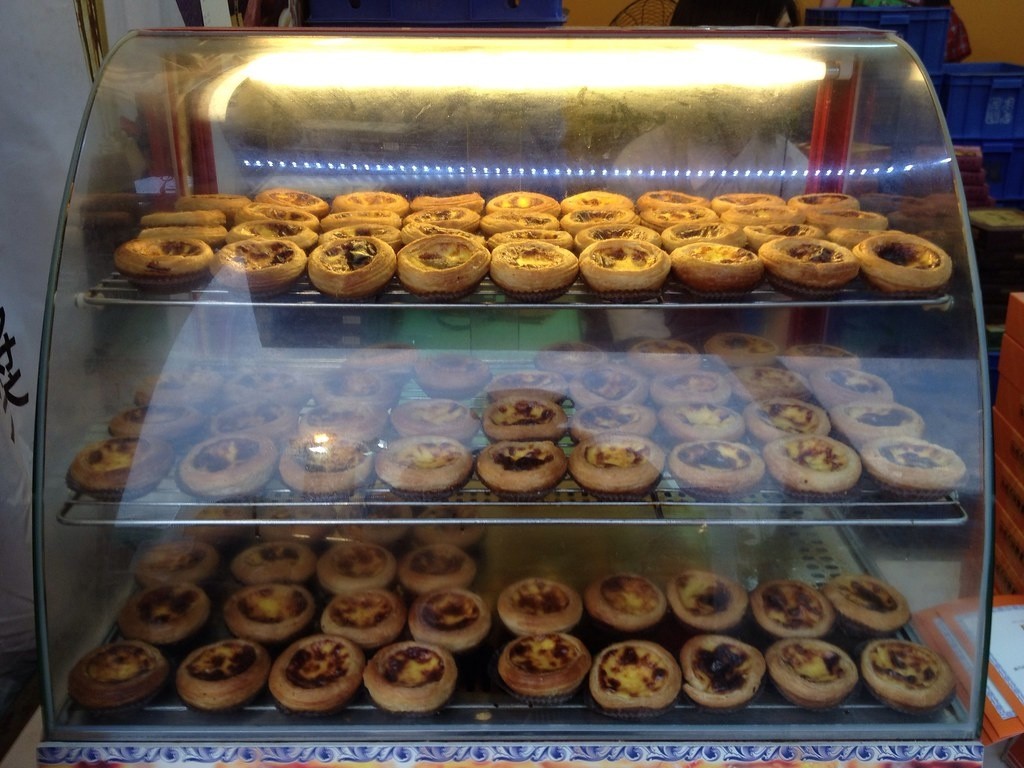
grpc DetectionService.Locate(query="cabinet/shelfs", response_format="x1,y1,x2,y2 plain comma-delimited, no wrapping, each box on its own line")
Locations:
35,27,994,739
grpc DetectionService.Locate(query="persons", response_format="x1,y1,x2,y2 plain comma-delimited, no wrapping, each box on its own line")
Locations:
600,1,809,351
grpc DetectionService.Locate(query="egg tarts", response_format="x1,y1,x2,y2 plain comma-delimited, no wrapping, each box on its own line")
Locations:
70,334,965,496
115,188,952,291
69,503,958,717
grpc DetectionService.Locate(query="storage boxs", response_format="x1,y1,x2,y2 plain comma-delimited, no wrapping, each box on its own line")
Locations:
958,292,1024,603
800,5,1024,203
302,0,568,29
